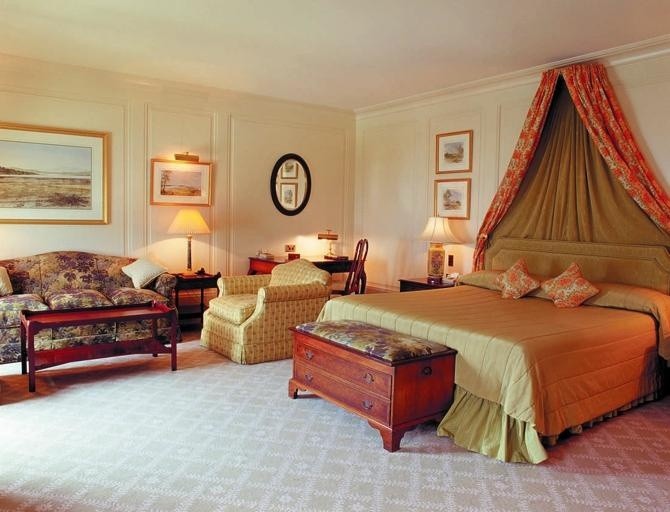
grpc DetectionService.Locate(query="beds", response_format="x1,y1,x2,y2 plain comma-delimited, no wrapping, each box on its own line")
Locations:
317,239,670,466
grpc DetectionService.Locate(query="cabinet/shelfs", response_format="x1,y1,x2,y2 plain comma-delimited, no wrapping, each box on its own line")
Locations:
288,320,458,453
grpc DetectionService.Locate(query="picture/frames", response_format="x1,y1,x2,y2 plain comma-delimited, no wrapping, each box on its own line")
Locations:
435,130,473,174
434,178,471,220
0,123,112,225
281,163,298,179
150,158,214,208
280,183,298,207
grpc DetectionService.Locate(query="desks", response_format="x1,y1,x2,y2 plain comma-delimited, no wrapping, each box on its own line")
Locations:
247,255,367,294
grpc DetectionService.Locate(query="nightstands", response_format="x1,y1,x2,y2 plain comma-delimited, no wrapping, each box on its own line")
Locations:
397,276,454,292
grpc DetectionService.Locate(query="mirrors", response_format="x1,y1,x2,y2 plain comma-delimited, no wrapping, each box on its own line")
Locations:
270,153,311,216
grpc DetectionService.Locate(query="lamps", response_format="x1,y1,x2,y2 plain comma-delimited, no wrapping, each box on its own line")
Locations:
415,217,464,284
317,229,338,259
166,209,212,271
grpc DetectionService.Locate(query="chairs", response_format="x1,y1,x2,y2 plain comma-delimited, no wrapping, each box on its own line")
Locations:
329,238,369,300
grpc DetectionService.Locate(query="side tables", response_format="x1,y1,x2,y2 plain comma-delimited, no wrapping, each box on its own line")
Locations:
170,267,221,330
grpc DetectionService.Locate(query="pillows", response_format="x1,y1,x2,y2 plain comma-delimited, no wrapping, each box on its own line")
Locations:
0,293,50,329
121,254,168,289
489,256,540,299
540,262,600,308
45,289,114,311
106,287,170,306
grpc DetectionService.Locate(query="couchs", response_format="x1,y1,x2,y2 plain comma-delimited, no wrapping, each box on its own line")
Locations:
200,259,333,365
0,251,182,365
